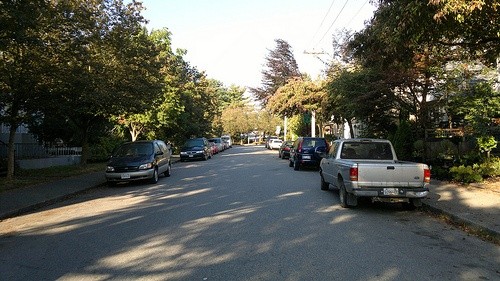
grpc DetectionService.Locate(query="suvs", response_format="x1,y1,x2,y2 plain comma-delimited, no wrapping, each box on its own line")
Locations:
179,137,212,162
289,137,331,170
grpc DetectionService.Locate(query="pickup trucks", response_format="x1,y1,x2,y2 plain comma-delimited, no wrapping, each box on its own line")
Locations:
319,138,431,208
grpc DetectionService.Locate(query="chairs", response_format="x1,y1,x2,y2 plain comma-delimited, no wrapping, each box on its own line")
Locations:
343,148,357,158
368,148,381,159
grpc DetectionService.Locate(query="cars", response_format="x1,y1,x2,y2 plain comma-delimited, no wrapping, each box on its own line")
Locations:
208,138,228,156
279,141,296,159
265,136,283,150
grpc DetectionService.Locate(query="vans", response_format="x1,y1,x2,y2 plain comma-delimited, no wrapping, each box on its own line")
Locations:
103,139,172,187
221,135,233,147
